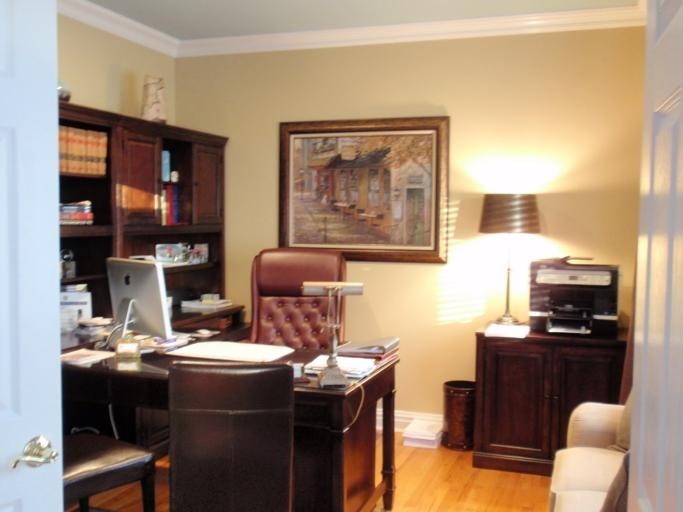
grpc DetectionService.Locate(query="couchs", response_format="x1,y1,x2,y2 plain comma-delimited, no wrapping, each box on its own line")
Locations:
546,387,628,512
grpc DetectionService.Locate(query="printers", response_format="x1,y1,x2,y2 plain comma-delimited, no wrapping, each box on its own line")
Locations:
529,261,619,334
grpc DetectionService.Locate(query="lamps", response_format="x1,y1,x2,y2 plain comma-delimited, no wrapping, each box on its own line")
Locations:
479,194,542,325
298,279,364,392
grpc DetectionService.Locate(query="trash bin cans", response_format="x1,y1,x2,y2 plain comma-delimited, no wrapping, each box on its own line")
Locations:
442,381,476,449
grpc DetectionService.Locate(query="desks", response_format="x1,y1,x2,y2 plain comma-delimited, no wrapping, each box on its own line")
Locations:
62,325,402,511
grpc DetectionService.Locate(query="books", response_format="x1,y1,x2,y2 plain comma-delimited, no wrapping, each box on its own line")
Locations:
59,127,183,226
303,327,400,379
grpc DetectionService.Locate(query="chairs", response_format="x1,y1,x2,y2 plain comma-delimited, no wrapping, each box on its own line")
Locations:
166,358,293,511
62,432,157,510
247,248,344,345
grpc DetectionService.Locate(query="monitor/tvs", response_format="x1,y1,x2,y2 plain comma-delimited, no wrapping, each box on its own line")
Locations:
106,257,172,341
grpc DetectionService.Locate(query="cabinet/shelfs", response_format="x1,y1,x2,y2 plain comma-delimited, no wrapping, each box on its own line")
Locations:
59,100,247,341
472,332,626,478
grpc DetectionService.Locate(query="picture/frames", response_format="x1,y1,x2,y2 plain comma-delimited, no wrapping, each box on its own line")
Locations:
276,115,450,264
